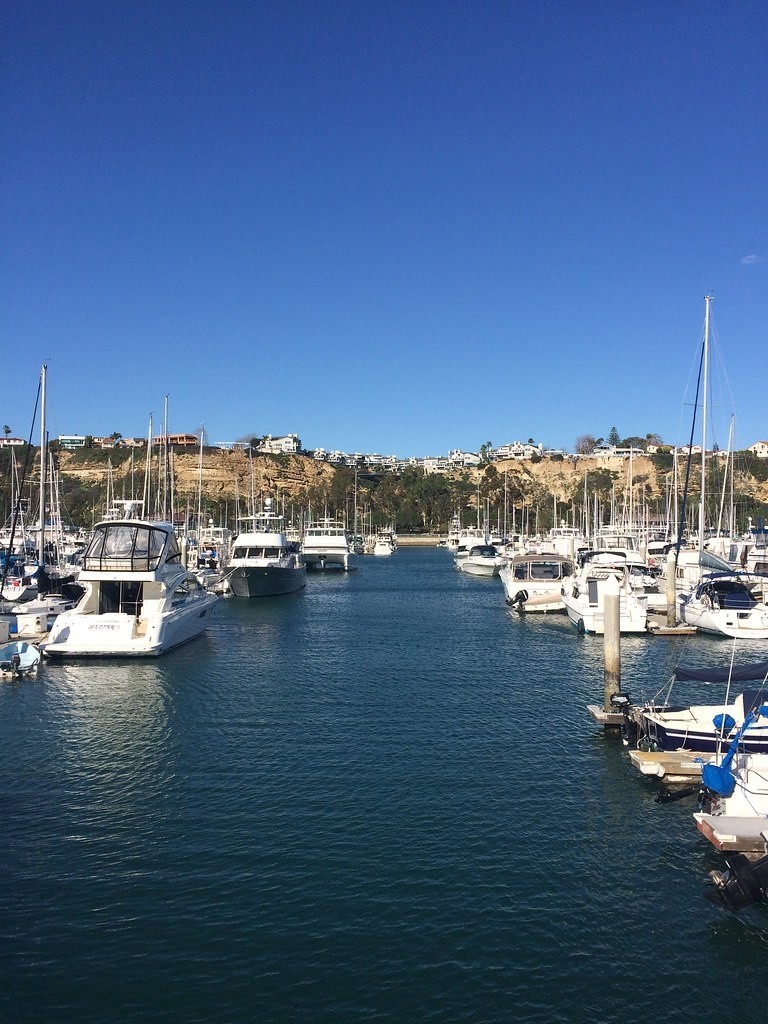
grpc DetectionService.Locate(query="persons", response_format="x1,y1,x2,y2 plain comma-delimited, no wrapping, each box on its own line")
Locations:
7,653,20,667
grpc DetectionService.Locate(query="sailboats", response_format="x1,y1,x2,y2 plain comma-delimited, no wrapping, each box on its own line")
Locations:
439,290,767,910
0,363,398,682
